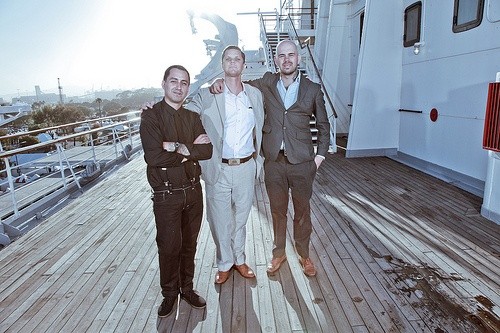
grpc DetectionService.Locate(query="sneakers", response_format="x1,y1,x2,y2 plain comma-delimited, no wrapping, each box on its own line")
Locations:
180,290,206,308
158,295,178,317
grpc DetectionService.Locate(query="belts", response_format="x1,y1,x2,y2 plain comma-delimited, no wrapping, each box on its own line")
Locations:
280,150,284,154
222,154,253,165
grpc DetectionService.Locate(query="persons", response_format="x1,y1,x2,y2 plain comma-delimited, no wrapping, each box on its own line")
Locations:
210,40,330,278
139,66,212,316
141,46,266,283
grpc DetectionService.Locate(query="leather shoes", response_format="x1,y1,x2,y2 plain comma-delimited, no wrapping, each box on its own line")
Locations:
215,266,232,283
266,254,287,273
232,264,255,278
299,256,316,276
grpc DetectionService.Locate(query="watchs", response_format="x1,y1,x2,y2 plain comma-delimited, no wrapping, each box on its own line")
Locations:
174,141,180,154
